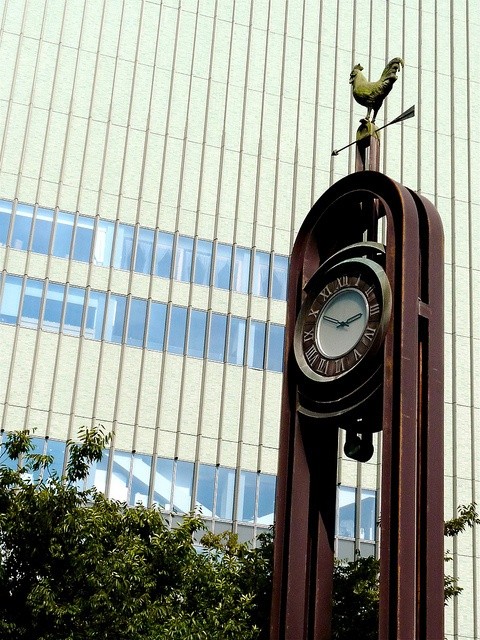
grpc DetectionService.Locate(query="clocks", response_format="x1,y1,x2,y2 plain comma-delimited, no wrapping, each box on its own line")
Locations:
293,256,393,384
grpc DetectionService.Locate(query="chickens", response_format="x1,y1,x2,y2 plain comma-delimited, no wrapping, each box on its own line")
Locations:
348,57,405,125
